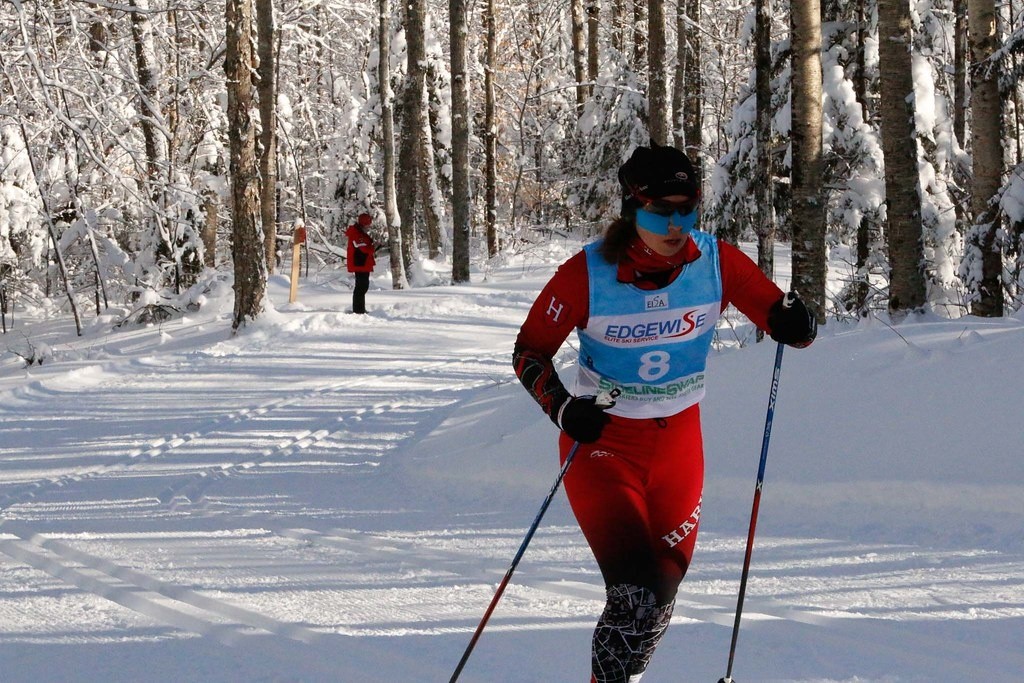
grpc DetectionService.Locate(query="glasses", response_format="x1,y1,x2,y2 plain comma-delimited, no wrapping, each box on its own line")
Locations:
638,198,699,217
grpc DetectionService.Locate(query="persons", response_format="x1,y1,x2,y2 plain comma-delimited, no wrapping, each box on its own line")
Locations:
512,137,818,683
344,213,385,314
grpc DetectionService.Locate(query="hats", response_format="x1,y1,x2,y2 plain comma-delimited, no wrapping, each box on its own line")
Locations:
618,138,697,200
358,213,372,227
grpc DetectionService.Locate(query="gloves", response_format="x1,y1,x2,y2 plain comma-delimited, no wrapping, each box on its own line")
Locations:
768,291,818,350
562,395,617,445
